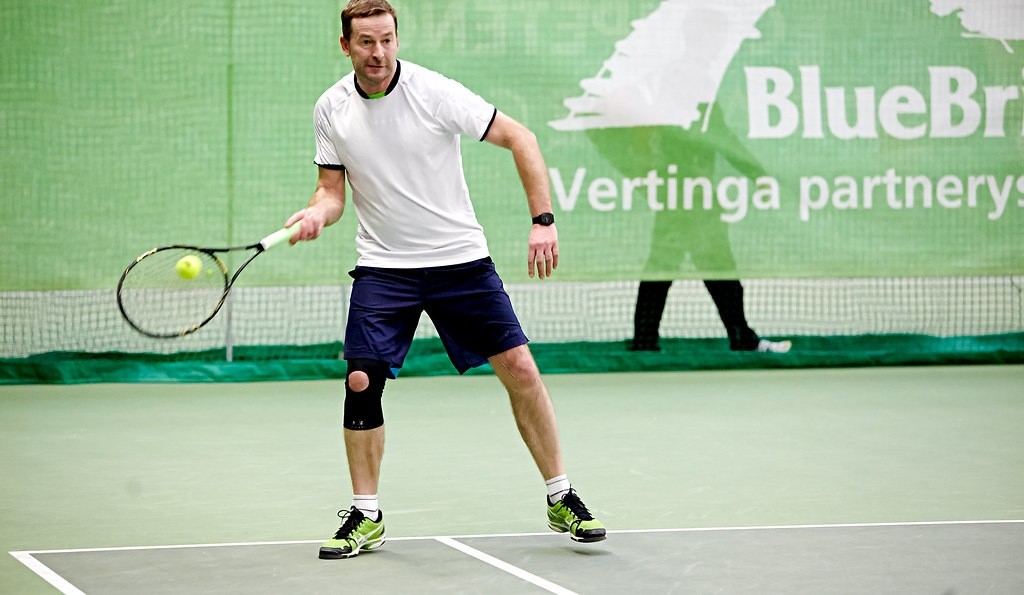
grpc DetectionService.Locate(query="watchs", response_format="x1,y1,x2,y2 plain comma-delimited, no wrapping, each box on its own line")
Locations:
532,211,555,226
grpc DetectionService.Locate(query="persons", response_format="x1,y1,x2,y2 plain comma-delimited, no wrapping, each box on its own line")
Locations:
626,102,793,352
279,1,607,560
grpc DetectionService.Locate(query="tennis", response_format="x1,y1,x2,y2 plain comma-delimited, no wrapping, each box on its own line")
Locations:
176,255,203,281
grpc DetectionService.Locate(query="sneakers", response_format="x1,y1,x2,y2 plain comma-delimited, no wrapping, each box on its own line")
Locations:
318,505,386,560
547,485,606,542
757,339,793,354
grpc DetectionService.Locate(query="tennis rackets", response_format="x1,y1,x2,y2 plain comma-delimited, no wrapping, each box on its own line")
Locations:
115,219,303,340
